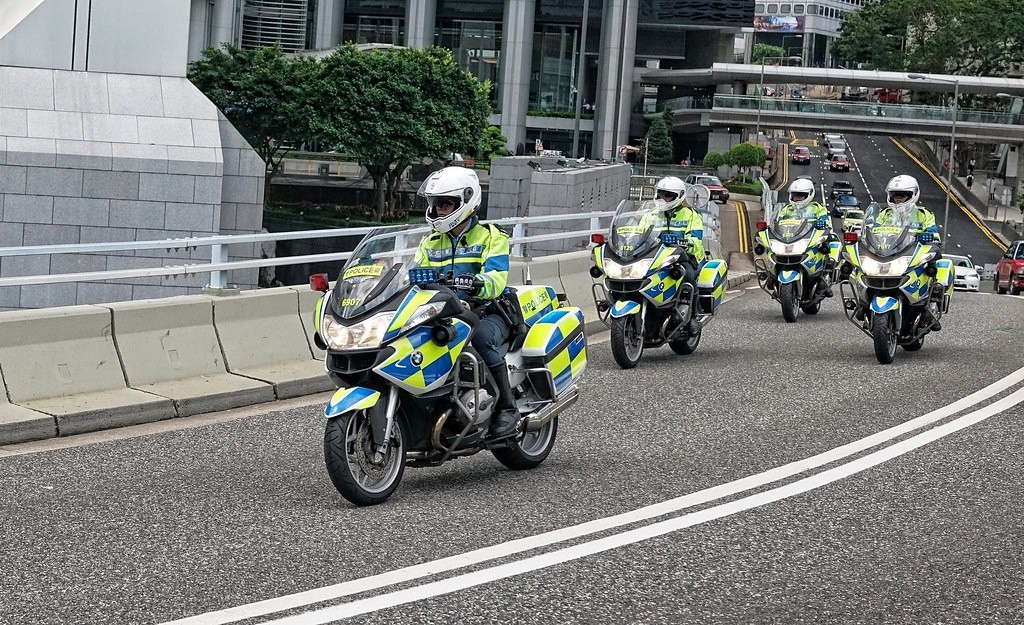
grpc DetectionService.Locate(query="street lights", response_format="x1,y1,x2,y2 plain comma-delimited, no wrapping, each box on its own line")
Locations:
784,45,809,99
776,34,802,98
908,73,961,253
753,55,802,181
885,33,905,106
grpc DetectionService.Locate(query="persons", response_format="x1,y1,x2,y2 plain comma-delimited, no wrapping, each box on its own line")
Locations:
873,174,941,332
771,178,832,298
637,176,704,333
764,87,767,95
990,177,995,200
945,160,950,169
779,89,783,96
966,173,973,189
970,157,975,171
681,157,697,166
413,165,521,437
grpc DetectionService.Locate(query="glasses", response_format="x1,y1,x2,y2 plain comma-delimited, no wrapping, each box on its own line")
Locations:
427,195,460,209
791,192,807,197
891,191,912,199
657,190,675,198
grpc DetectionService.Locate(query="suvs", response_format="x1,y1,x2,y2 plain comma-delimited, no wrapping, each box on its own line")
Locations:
993,239,1024,295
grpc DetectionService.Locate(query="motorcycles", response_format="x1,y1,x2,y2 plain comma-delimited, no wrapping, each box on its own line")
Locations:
588,198,728,369
839,201,955,364
752,201,844,323
307,223,589,508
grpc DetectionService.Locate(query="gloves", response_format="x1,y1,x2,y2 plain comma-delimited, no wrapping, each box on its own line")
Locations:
676,237,689,247
813,219,826,230
454,273,477,291
919,232,934,245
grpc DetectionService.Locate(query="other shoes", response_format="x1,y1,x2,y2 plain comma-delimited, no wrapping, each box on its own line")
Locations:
768,278,778,289
922,312,942,331
816,278,833,297
856,307,871,321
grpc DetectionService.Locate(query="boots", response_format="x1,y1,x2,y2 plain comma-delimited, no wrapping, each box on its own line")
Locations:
686,288,700,336
489,359,521,436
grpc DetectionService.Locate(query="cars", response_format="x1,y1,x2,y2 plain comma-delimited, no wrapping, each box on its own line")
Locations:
448,152,474,166
822,131,865,253
795,174,817,189
757,85,916,119
791,146,813,165
683,172,730,204
941,253,984,292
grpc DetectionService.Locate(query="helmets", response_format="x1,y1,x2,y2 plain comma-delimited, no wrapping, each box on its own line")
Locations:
417,166,481,234
654,177,686,212
884,175,921,213
787,179,815,209
